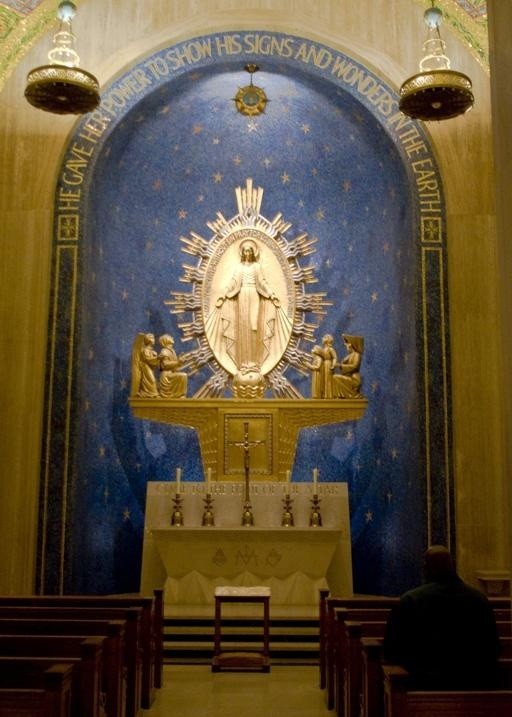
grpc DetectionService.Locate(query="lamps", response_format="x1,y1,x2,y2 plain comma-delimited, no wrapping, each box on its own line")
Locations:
23,0,101,118
396,0,476,122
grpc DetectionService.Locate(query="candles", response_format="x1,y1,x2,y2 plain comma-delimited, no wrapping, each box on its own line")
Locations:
285,467,291,495
314,465,317,494
176,466,180,494
207,466,212,494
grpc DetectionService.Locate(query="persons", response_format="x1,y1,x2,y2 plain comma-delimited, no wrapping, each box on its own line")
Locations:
323,334,337,398
205,245,294,370
384,546,504,691
137,333,165,399
234,441,261,470
332,333,364,398
158,334,188,398
304,345,324,399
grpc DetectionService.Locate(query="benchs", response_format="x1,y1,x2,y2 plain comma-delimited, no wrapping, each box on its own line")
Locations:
0,588,165,717
319,587,512,717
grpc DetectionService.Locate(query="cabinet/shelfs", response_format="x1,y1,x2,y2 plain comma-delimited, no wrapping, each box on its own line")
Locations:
211,586,271,673
